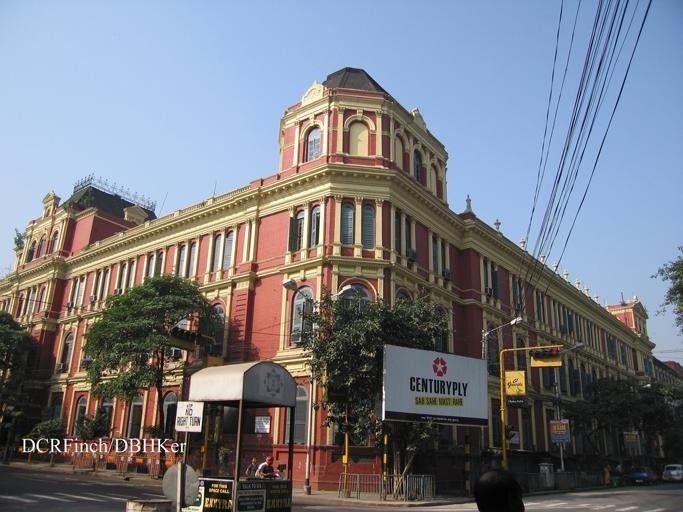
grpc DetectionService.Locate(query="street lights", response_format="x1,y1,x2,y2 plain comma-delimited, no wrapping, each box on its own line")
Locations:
480,316,524,448
282,278,356,486
554,342,586,472
630,383,651,394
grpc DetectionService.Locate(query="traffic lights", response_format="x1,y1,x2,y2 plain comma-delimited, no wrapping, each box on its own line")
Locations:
169,326,196,343
504,424,516,441
529,347,559,359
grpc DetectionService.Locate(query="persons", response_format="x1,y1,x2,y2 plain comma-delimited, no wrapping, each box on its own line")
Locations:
471,467,525,511
244,457,257,478
252,455,281,480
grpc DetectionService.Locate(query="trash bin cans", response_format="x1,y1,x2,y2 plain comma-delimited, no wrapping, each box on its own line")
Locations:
538,463,555,491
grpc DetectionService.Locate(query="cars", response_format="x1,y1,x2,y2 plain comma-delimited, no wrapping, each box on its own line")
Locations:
661,464,683,483
630,467,661,486
